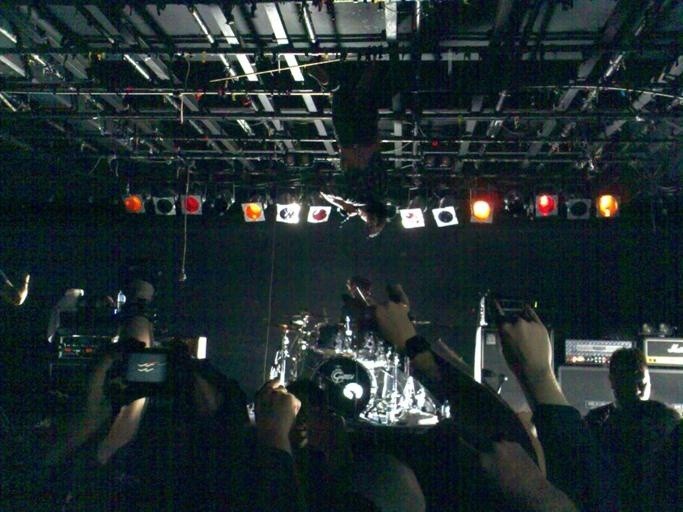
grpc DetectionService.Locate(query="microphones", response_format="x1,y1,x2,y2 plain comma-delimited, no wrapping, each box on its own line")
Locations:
497,375,509,395
334,373,354,381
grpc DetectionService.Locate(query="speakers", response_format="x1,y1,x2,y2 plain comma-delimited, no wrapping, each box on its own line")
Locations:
557,364,614,418
649,369,683,408
473,326,555,416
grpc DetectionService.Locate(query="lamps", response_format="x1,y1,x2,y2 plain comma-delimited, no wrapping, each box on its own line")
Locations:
120,182,336,228
394,186,626,232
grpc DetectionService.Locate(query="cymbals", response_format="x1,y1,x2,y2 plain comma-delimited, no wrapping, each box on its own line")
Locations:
264,319,302,331
409,312,433,327
281,312,330,318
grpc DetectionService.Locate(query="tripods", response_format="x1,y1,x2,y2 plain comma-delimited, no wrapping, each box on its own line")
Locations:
361,347,437,421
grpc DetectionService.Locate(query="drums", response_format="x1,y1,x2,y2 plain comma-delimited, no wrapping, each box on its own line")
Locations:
313,322,347,357
309,353,379,418
302,349,328,381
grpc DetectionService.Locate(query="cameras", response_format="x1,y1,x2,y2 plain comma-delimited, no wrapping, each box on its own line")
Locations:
121,348,174,386
